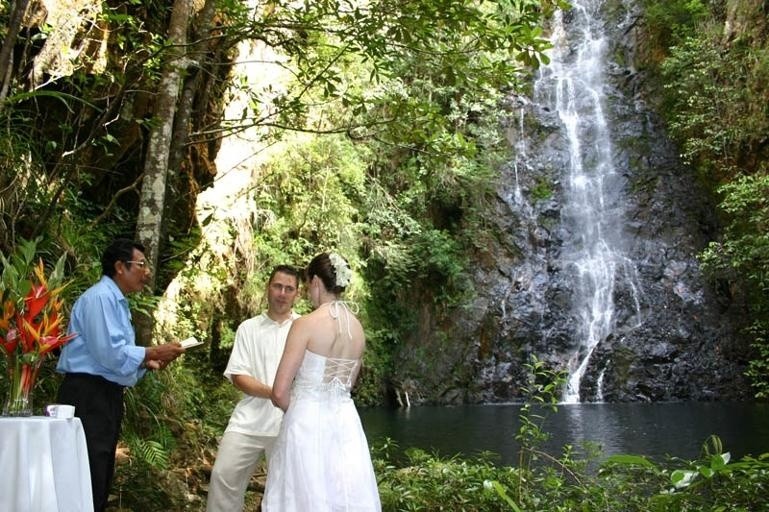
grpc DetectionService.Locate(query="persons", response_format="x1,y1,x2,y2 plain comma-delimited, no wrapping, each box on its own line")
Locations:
261,252,383,511
53,242,185,512
206,267,301,512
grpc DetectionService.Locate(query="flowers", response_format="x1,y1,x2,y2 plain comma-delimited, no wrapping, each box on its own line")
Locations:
0,232,81,409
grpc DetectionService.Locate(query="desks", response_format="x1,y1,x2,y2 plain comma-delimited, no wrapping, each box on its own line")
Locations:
0,415,95,512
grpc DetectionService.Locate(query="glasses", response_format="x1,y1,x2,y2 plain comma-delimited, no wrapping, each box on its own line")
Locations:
126,260,151,269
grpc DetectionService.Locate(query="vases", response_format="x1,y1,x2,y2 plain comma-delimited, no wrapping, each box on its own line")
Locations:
4,350,43,417
